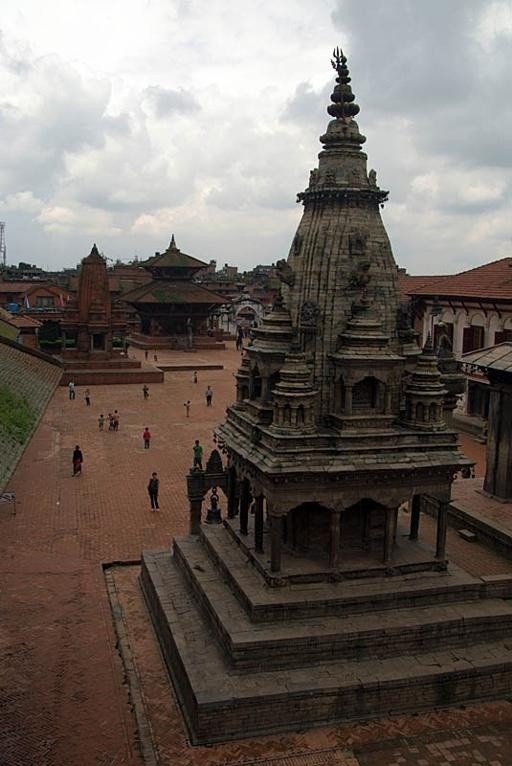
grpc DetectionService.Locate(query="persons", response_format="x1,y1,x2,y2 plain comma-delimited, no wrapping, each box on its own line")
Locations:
84,388,91,408
194,371,198,383
68,379,76,400
147,472,160,512
143,385,149,400
145,349,149,361
192,440,204,470
98,410,120,431
143,427,151,448
154,349,158,361
205,385,213,406
72,445,83,477
